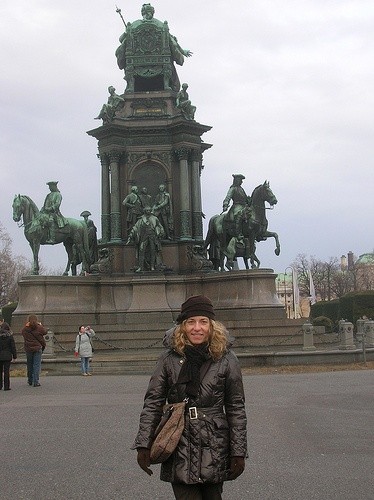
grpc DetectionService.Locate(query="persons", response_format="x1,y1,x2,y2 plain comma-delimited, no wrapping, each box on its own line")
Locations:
93,86,124,120
151,184,174,241
223,174,249,239
121,185,143,234
22,314,47,387
74,325,98,376
139,188,153,207
114,3,193,92
179,83,196,118
130,295,248,500
126,206,165,272
80,211,97,263
0,322,18,390
40,181,62,246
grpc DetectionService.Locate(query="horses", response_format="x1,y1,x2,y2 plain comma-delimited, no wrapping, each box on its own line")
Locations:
12,194,92,277
201,180,282,273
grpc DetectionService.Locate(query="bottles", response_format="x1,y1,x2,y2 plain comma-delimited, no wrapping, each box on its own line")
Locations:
84,327,89,330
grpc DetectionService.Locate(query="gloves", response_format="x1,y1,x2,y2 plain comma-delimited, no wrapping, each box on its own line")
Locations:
74,352,78,358
13,354,17,359
137,448,153,476
86,326,90,330
229,456,245,480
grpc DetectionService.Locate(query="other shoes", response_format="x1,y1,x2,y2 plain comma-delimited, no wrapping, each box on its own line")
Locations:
87,372,92,376
34,382,40,386
4,388,11,390
29,382,32,385
82,372,87,376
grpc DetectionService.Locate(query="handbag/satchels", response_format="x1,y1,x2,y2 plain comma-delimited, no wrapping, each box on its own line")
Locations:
42,346,45,353
92,348,94,352
149,403,187,464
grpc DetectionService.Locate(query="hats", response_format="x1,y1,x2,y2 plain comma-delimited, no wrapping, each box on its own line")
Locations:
181,295,215,322
